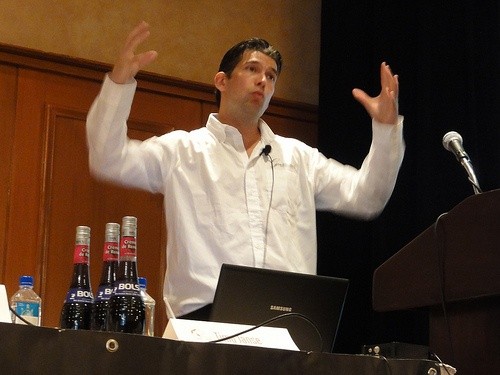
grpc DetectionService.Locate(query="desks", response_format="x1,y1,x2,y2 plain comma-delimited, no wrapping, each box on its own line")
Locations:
0,322,441,375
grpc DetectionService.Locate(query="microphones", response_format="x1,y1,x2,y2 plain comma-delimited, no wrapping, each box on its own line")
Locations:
261,145,272,155
443,131,471,164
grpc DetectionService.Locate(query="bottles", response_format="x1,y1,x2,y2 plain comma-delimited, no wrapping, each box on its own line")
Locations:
103,216,147,335
138,277,156,337
60,225,94,330
90,223,120,330
9,275,43,327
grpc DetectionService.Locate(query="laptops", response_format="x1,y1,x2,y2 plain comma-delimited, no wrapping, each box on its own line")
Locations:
207,263,351,354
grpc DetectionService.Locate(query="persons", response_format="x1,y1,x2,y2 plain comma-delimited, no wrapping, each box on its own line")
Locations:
86,19,404,327
